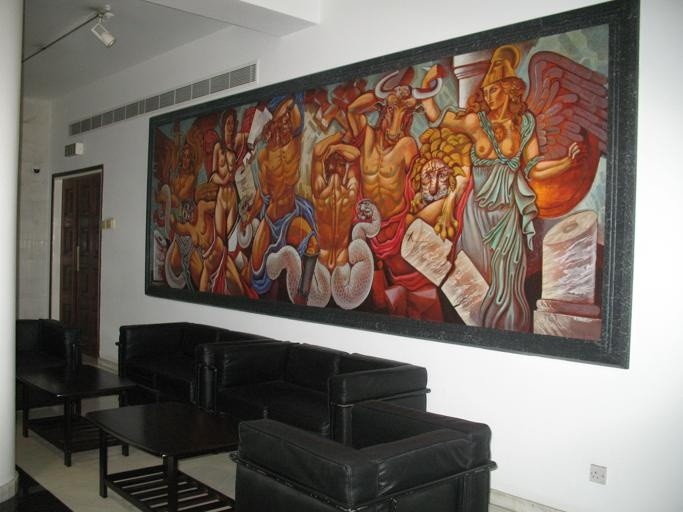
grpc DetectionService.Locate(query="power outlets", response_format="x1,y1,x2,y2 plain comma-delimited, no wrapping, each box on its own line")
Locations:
590,464,607,485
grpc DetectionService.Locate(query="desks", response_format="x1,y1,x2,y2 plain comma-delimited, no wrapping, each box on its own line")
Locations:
87,402,240,512
16,364,139,468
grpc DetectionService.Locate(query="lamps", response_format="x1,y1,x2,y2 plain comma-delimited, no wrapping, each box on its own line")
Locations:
92,6,116,48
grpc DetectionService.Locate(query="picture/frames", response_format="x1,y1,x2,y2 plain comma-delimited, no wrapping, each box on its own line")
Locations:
144,0,640,369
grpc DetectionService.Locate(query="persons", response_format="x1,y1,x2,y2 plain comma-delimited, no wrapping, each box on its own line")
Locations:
151,41,583,339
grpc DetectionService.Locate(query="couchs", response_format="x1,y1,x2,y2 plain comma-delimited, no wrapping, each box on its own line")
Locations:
115,322,434,452
15,319,84,409
228,397,499,512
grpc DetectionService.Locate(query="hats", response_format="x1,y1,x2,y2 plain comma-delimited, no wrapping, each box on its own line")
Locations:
480,56,517,90
